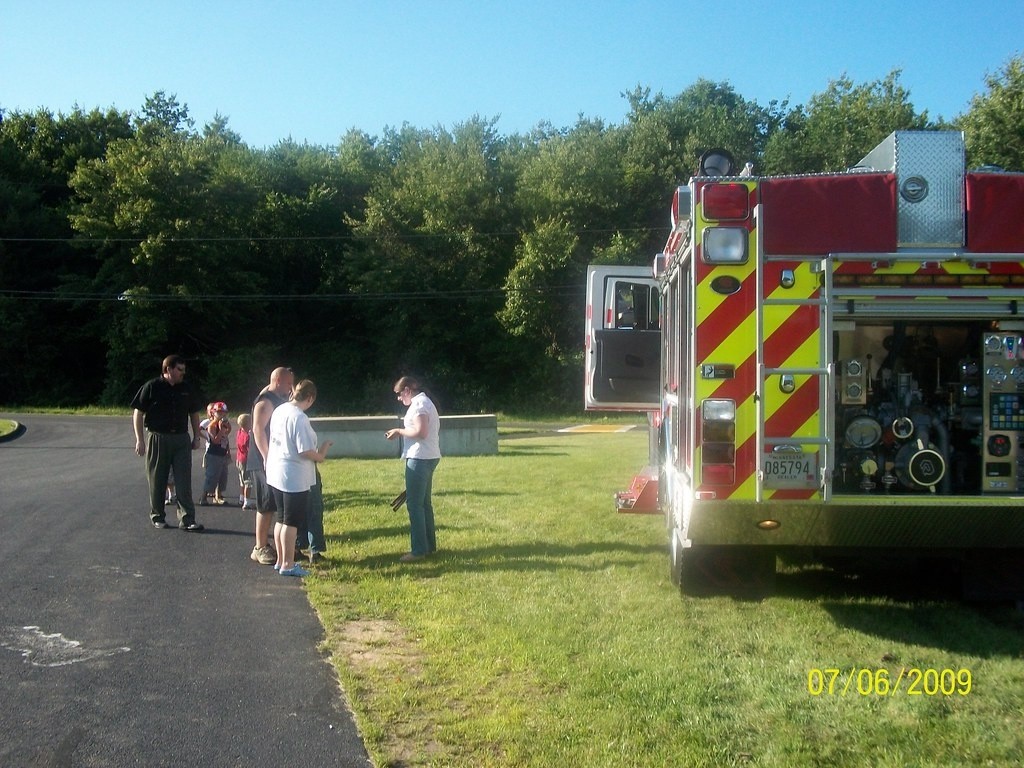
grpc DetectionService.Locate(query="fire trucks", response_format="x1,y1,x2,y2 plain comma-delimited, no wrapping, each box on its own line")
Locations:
583,133,1024,610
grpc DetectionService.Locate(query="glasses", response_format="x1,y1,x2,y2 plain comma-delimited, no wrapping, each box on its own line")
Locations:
394,389,405,397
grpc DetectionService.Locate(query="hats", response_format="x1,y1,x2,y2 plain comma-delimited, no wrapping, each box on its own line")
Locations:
213,401,228,412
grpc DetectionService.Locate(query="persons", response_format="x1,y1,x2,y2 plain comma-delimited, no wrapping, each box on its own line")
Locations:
199,402,232,505
165,462,176,505
235,414,257,509
245,367,294,564
199,403,215,497
384,376,442,561
130,356,205,532
264,378,324,576
294,440,335,563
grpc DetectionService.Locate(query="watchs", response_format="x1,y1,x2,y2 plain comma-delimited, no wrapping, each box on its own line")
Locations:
194,438,200,440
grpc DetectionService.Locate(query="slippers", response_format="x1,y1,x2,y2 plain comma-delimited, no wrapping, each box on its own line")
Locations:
274,562,302,570
279,566,310,576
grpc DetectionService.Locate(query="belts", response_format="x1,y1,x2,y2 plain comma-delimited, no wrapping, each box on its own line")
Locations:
148,429,187,434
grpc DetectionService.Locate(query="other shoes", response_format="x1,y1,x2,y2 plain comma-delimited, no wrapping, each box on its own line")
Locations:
239,500,244,505
294,551,309,562
310,553,325,564
426,552,431,555
212,497,226,505
242,503,257,510
168,497,175,504
400,553,425,561
199,498,208,505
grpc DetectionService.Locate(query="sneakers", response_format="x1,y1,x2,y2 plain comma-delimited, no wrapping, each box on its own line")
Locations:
250,544,276,564
178,520,204,532
149,509,169,529
